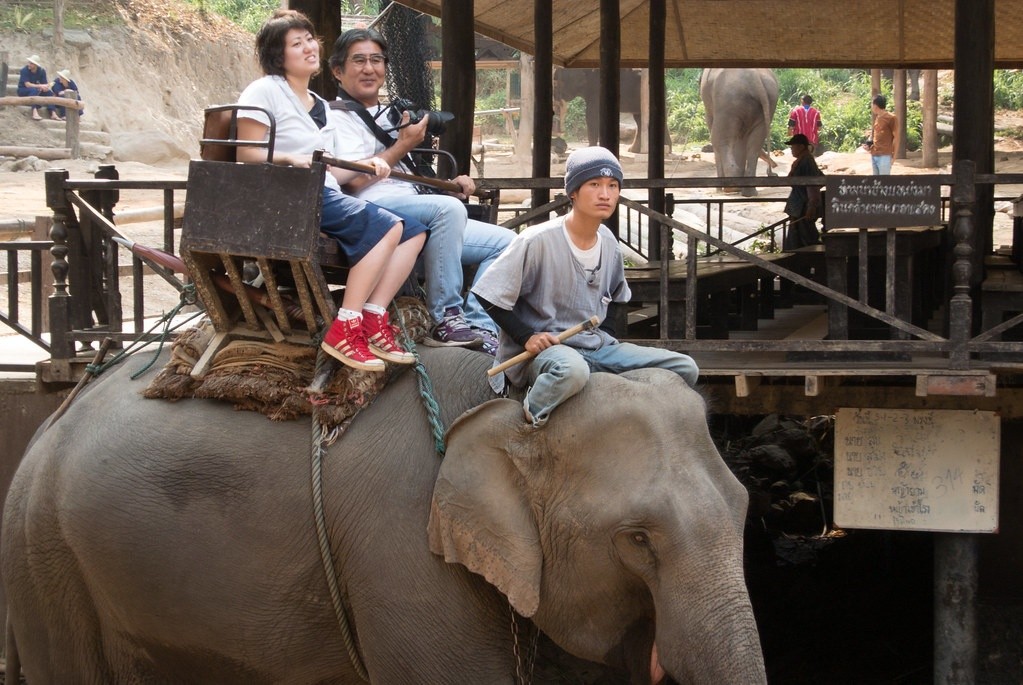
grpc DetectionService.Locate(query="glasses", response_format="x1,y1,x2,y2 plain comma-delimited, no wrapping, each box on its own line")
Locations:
347,54,386,66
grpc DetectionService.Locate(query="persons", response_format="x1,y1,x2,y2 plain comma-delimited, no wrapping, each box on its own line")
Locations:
17,55,62,121
235,10,431,371
52,69,84,123
322,27,519,356
783,133,826,252
788,95,823,157
470,146,699,428
863,94,900,176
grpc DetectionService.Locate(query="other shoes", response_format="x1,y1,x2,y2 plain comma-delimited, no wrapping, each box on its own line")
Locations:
32,115,42,120
51,116,62,121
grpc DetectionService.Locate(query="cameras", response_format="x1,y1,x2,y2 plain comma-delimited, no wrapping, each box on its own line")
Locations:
386,98,454,137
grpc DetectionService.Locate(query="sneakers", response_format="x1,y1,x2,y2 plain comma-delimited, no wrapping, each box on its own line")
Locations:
362,311,415,364
321,316,385,371
471,327,499,356
424,306,484,349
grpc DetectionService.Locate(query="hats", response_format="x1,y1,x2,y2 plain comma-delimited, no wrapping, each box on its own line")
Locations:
57,70,72,83
564,146,624,201
27,55,41,67
783,135,810,146
873,95,887,110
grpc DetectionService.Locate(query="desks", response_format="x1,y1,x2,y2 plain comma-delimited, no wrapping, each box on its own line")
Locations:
782,245,829,305
980,269,1023,342
678,252,797,320
984,254,1017,270
787,225,946,364
621,262,759,340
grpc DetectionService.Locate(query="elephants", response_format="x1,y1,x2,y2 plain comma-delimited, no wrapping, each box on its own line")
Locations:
554,66,674,155
699,67,779,199
1,328,771,685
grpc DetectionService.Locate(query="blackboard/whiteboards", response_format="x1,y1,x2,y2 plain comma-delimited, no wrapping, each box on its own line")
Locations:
833,407,1001,533
824,176,941,231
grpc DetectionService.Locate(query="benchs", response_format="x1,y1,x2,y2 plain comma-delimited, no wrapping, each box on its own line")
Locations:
180,104,501,269
782,312,828,340
627,305,659,332
